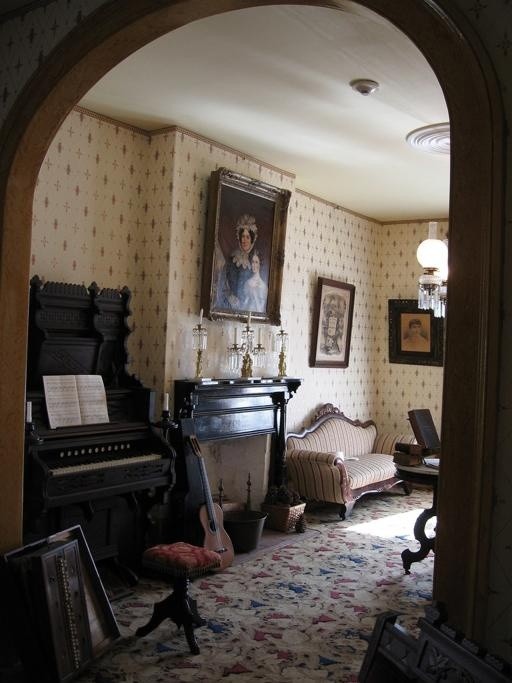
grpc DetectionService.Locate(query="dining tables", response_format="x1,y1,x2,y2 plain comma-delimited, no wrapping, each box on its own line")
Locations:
260,483,307,532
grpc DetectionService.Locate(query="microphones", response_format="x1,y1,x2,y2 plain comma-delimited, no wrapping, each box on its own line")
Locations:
134,541,222,656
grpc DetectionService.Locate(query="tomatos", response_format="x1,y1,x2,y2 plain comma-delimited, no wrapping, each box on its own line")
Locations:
23,274,176,588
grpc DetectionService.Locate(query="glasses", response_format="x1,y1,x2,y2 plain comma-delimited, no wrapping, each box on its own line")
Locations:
417,220,448,319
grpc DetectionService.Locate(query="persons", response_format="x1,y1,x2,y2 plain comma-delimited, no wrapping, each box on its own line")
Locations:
212,212,267,314
399,317,430,352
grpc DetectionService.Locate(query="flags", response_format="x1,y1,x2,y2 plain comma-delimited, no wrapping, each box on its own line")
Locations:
223,509,268,554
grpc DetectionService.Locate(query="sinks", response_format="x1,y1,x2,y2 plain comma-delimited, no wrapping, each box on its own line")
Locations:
285,403,419,520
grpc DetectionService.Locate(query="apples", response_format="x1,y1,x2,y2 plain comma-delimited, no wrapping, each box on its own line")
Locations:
163,392,169,411
26,401,32,422
199,308,204,327
280,320,284,329
247,310,252,330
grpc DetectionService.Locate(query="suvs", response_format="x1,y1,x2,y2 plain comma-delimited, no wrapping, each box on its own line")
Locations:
222,509,269,555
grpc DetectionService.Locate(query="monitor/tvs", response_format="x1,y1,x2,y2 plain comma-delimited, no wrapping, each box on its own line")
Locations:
42,374,110,430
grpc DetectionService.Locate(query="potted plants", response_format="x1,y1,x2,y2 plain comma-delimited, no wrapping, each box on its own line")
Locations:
388,298,444,367
200,166,292,327
309,276,356,368
